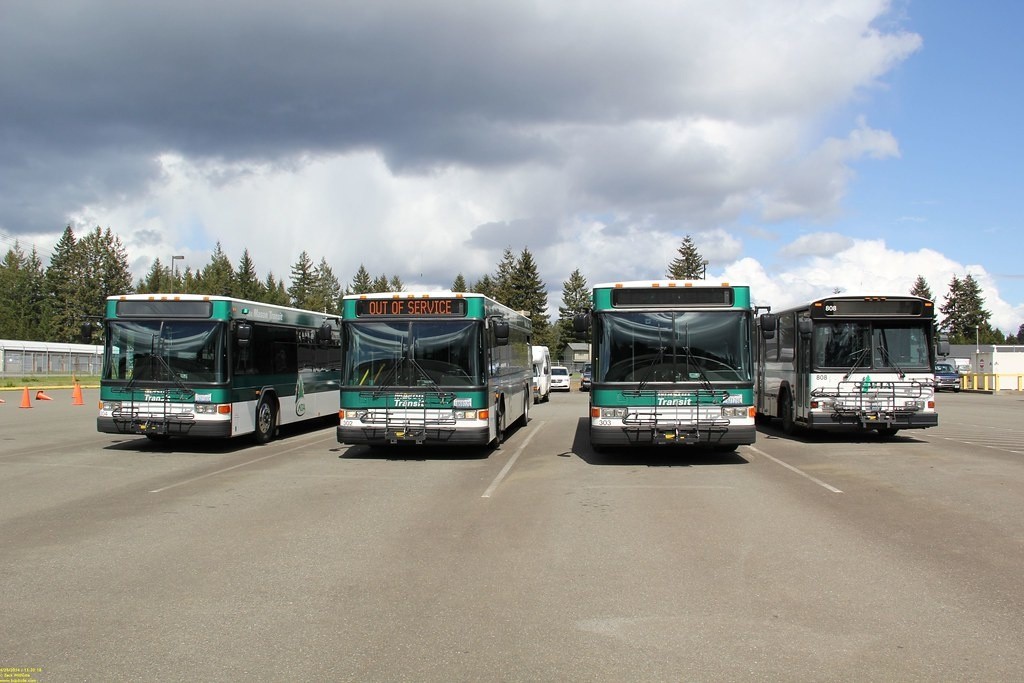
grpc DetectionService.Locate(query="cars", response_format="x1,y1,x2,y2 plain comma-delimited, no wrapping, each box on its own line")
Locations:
551,367,573,393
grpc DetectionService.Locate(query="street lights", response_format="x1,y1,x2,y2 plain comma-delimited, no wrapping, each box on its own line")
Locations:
170,255,184,294
701,259,709,280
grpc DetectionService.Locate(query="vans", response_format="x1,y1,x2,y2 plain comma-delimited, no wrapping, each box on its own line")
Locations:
530,346,553,403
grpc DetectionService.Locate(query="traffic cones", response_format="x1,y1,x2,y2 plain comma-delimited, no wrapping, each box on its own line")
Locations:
18,385,32,408
72,383,78,398
0,399,5,403
72,385,85,404
36,390,53,400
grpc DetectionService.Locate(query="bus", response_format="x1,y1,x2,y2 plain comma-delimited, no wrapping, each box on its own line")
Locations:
97,293,341,444
336,291,534,448
749,296,950,439
571,280,778,458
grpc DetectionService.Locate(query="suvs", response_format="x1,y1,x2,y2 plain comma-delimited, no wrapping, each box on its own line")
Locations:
934,364,960,393
580,361,592,391
